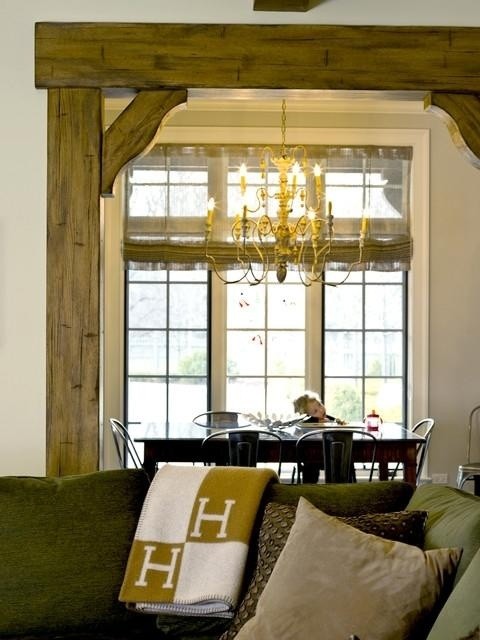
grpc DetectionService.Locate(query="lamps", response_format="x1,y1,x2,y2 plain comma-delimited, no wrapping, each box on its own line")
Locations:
202,98,368,286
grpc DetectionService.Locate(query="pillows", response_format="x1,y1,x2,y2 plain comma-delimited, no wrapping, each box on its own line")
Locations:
219,496,463,640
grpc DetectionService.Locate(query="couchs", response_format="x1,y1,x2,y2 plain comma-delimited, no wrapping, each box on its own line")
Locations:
1,470,479,640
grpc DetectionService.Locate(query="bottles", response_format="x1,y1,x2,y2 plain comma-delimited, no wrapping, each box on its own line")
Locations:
367,409,381,432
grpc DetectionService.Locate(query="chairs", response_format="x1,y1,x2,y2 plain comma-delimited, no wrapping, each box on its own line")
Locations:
458,405,480,491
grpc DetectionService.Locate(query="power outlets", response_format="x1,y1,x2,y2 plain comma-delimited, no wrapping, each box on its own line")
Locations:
433,474,448,484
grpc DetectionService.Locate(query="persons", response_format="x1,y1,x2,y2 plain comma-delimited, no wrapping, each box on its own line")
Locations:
295,391,358,485
